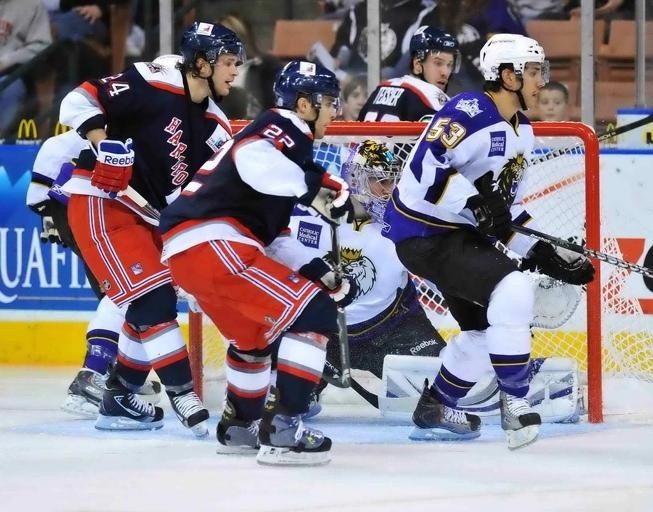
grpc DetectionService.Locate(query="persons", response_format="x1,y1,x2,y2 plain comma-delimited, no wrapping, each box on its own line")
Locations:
264,140,447,420
26,54,185,408
47,20,245,429
380,33,595,435
1,2,629,142
159,58,350,451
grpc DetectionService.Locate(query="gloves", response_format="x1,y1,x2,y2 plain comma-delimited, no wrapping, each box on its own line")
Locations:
527,240,595,284
301,257,358,306
301,166,354,225
89,138,135,198
474,171,512,243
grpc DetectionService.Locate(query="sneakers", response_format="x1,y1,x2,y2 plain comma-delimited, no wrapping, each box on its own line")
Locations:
260,408,332,451
413,378,481,433
216,417,259,450
66,368,164,422
167,389,209,427
498,390,540,431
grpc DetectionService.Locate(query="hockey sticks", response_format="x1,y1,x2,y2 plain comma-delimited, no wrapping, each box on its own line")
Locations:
322,190,353,387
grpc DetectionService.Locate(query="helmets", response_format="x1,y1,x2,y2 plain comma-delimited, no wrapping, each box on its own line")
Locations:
273,60,340,135
179,20,248,103
480,34,551,111
409,26,462,94
352,140,403,219
